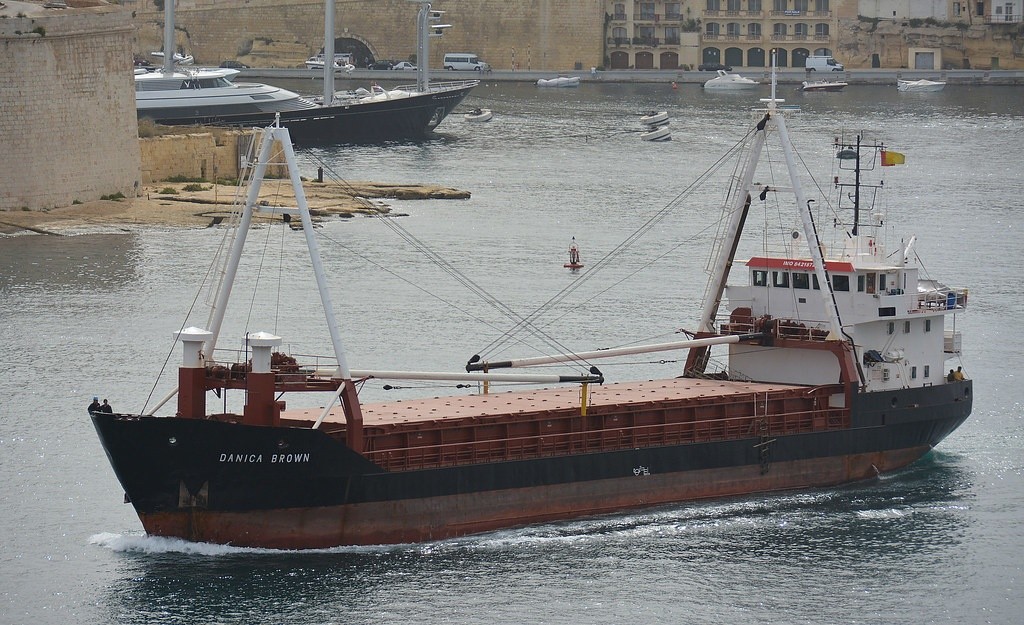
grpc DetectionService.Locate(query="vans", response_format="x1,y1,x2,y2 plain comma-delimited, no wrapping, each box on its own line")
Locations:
443,52,486,71
805,54,843,73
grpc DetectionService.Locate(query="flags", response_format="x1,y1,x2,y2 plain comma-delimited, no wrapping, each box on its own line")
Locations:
880,150,905,165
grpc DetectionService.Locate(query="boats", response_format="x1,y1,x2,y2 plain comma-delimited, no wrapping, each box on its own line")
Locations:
85,44,974,552
800,80,849,91
537,75,580,87
126,0,241,90
133,1,481,143
896,78,946,92
703,68,760,92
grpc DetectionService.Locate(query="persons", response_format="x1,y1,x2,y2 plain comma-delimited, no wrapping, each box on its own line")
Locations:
953,365,965,383
87,396,112,413
947,368,955,382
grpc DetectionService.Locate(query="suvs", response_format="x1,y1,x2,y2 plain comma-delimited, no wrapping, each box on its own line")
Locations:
305,60,355,77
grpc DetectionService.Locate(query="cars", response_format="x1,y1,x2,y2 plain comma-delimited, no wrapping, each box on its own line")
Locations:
697,61,733,72
393,62,421,72
133,58,149,66
368,59,396,71
218,60,250,69
